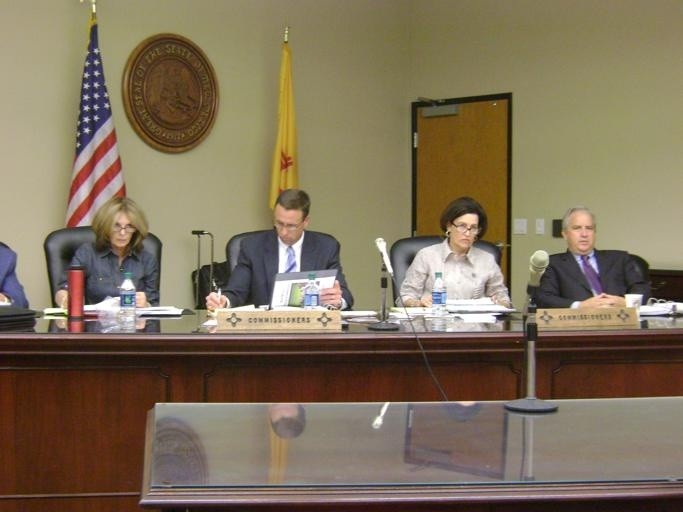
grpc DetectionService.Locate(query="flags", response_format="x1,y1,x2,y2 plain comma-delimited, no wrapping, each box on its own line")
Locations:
269,44,297,209
65,15,129,229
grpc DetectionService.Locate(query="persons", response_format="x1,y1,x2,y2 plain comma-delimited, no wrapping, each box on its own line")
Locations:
537,207,651,309
0,243,30,307
205,190,353,313
396,197,510,307
54,197,160,308
266,403,305,440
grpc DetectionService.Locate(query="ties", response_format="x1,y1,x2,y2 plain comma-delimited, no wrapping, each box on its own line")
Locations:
284,247,296,273
581,255,602,296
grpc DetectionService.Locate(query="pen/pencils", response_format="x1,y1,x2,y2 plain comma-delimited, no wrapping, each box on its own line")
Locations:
216,288,221,303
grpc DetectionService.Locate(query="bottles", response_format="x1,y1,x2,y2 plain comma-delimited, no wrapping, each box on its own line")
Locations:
119,273,137,330
431,272,447,326
67,266,87,319
303,273,321,312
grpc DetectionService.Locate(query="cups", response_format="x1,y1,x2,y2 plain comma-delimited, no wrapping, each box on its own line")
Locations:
624,293,644,319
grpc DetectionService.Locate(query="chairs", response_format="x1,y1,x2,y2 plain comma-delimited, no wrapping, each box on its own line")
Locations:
630,255,650,274
390,235,503,308
227,230,273,280
44,226,163,306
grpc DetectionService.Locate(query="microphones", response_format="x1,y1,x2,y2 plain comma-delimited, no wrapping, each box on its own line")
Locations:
374,237,395,276
522,251,550,302
371,402,390,430
191,230,214,238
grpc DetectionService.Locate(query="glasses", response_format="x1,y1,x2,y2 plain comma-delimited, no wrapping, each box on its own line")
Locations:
450,222,482,236
270,219,302,231
109,225,137,233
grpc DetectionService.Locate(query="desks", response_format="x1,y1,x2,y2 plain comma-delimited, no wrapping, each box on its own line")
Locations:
140,395,682,512
2,296,682,512
